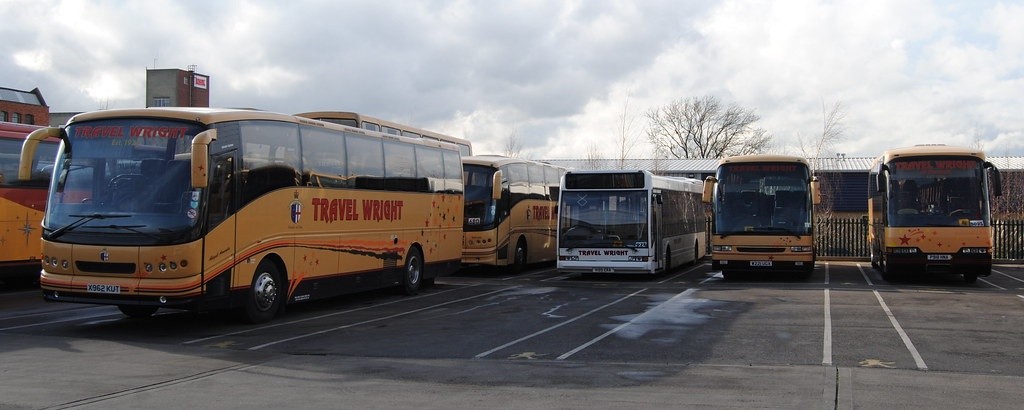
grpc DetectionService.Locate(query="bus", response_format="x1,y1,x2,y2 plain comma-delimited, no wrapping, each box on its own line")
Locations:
456,156,566,274
288,111,473,167
865,146,1003,284
552,168,710,278
700,156,821,282
17,106,465,327
0,122,166,267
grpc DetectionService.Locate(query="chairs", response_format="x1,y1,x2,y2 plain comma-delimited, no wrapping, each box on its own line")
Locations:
620,200,631,216
136,159,191,196
742,190,794,218
